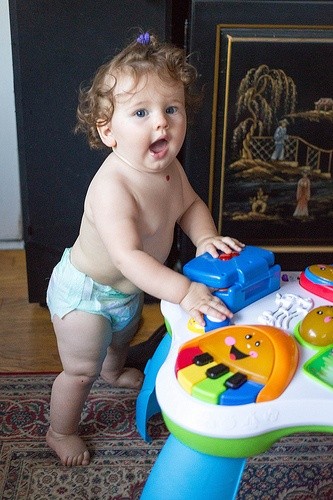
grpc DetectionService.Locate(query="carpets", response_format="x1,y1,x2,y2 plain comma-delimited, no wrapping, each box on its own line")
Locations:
0,369,333,499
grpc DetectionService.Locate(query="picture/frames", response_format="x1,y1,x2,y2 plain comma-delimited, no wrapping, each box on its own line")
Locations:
208,23,332,250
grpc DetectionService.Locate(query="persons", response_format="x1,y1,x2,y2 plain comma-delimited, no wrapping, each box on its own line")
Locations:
46,32,245,467
